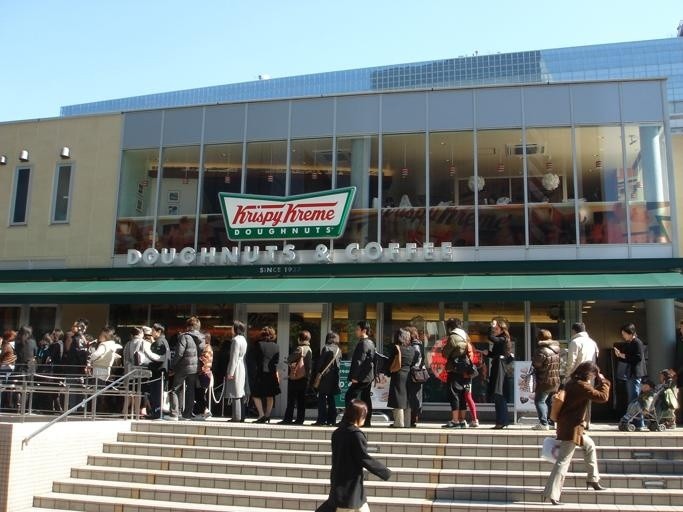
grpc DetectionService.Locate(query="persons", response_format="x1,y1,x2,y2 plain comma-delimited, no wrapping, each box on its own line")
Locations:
1,317,250,421
440,319,682,432
329,399,393,512
248,320,427,427
544,360,610,504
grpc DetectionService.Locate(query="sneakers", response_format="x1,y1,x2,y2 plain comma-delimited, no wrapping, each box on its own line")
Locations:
276,419,304,424
441,419,480,429
311,418,336,426
146,408,212,421
531,422,557,430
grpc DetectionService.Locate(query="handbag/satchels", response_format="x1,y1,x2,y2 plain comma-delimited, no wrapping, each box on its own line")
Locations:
449,345,479,378
389,354,402,373
312,372,322,389
410,365,431,383
288,357,306,380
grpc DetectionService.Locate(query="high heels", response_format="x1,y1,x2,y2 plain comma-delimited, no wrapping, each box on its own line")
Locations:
544,497,565,505
490,424,508,429
587,481,606,490
253,416,270,423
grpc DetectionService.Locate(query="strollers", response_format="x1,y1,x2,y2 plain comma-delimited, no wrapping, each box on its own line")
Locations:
618,376,680,432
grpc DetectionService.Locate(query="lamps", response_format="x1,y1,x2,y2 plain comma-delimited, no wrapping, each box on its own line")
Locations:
19,150,29,162
60,147,70,159
0,156,7,165
